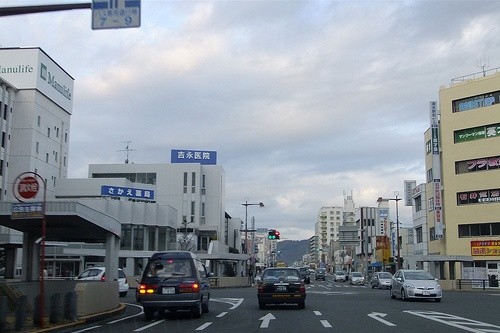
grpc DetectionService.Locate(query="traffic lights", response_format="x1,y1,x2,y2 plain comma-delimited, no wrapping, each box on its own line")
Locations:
268,235,279,239
269,232,279,235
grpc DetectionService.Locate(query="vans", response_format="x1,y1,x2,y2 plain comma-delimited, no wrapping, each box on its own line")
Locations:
139,251,214,318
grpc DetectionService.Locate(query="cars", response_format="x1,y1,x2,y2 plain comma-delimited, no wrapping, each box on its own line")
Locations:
73,267,129,296
297,265,348,284
350,272,365,286
370,272,393,289
257,267,306,309
390,269,442,301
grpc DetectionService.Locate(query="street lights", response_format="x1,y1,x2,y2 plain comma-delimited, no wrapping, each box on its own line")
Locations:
377,196,402,270
239,201,264,254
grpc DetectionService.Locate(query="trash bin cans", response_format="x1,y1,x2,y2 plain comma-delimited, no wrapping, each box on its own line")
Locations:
488,273,499,287
